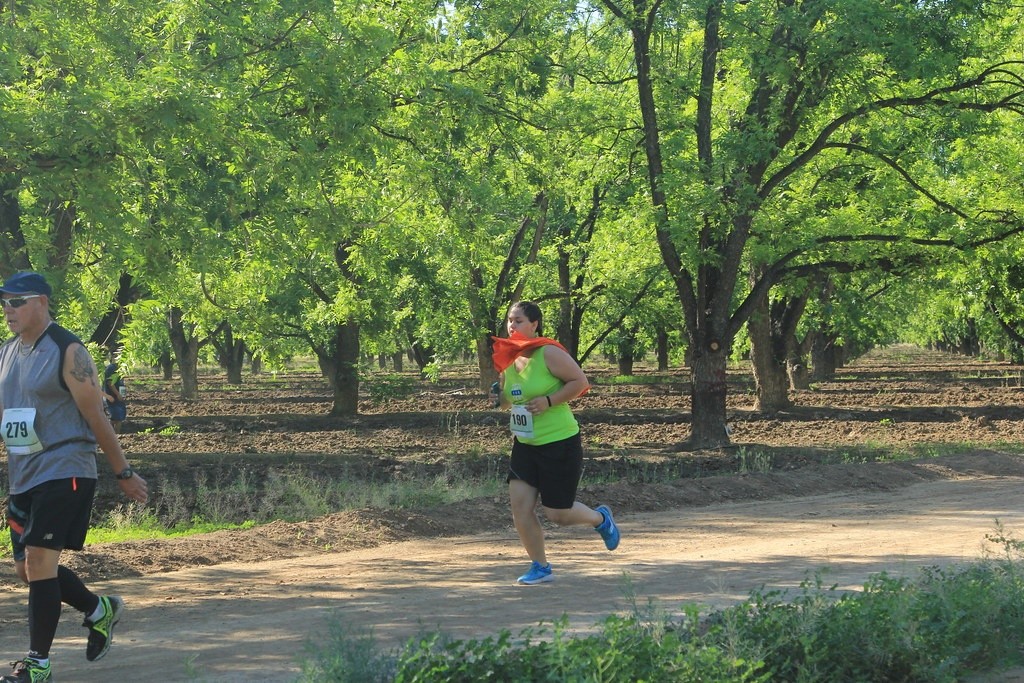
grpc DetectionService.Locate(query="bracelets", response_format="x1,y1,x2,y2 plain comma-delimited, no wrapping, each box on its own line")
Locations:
546,396,552,407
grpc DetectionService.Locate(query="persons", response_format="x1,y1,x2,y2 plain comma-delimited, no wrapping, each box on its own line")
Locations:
0,272,149,683
489,302,619,585
100,352,127,433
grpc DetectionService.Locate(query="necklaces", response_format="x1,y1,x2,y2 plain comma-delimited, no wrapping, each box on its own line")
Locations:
19,321,53,358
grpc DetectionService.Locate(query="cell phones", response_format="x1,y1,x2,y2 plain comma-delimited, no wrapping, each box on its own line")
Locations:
492,382,500,409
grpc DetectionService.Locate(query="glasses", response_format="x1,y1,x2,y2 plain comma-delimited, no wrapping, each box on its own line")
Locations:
0,295,42,308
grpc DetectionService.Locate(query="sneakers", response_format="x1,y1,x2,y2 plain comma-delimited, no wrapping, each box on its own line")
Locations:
593,505,620,551
0,657,53,683
82,595,124,661
517,562,554,584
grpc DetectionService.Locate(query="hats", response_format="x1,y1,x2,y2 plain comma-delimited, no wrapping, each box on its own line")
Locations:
0,272,51,298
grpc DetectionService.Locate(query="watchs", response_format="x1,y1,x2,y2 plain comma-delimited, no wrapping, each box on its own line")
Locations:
116,466,133,480
